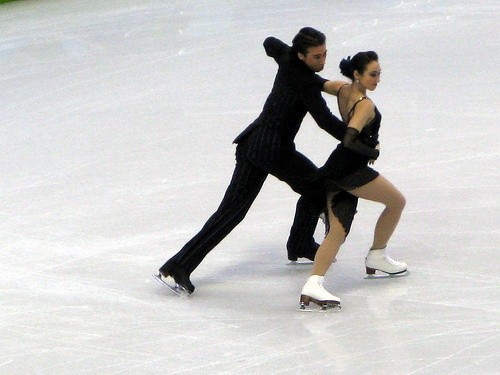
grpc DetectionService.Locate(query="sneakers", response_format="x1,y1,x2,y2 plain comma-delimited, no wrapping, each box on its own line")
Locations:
363,244,410,279
297,274,343,313
286,241,337,265
151,261,195,299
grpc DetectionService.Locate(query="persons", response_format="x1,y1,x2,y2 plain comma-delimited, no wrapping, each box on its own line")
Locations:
299,51,407,309
158,27,380,295
